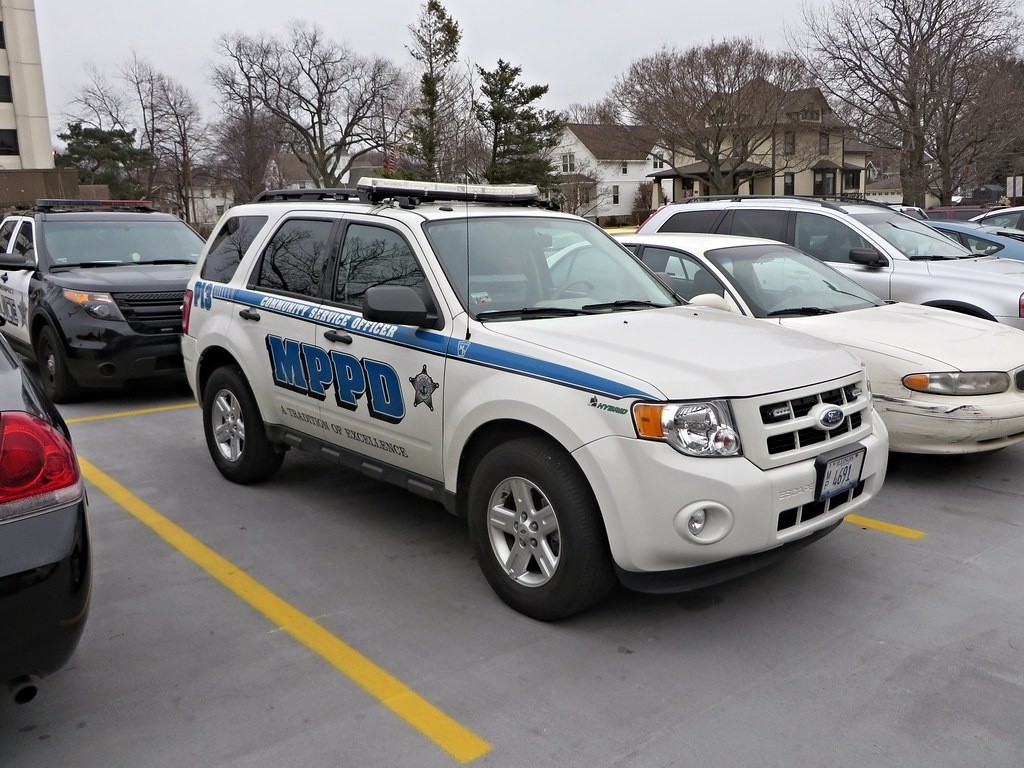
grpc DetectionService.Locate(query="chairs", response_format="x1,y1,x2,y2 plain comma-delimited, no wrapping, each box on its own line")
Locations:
655,265,724,300
798,221,852,263
